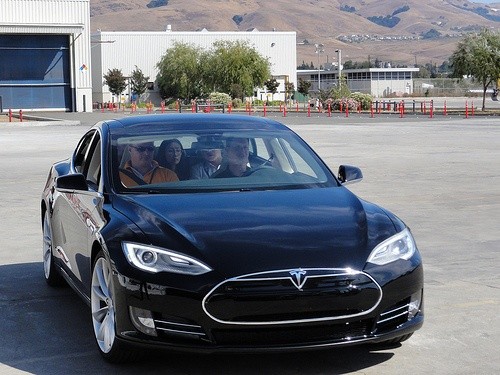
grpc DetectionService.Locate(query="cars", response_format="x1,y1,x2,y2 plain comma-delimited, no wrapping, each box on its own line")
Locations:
38,120,417,367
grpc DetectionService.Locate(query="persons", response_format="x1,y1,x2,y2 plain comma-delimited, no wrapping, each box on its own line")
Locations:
207,137,254,178
118,139,179,187
188,137,225,179
155,139,186,183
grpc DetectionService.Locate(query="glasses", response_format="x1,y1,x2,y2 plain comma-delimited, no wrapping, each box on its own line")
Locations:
130,145,156,153
